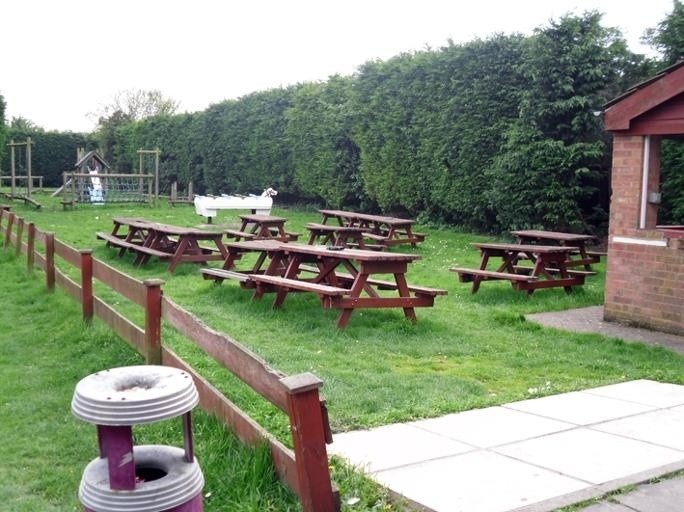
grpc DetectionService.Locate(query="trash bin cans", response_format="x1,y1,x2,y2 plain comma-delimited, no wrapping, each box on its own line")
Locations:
72,365,206,511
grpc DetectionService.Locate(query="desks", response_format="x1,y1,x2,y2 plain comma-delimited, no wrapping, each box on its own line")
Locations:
350,213,416,251
318,209,361,238
272,242,435,335
233,214,288,243
106,217,149,258
194,194,273,224
